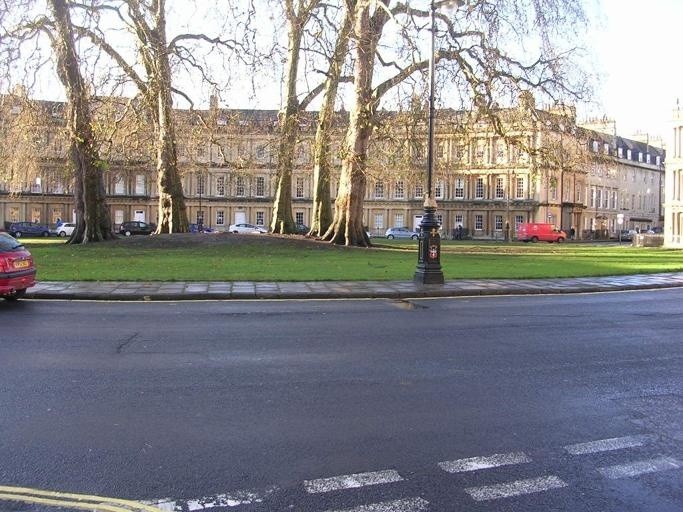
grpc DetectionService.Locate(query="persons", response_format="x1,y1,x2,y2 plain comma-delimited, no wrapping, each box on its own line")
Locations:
455,225,462,240
570,228,575,240
56,218,60,223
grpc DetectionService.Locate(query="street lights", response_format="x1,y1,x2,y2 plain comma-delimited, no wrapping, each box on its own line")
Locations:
417,0,466,269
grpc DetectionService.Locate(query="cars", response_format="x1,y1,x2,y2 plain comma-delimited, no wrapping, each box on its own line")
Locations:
229,223,267,233
619,230,637,241
297,225,309,234
190,224,213,233
56,223,76,237
10,221,52,237
385,228,419,240
0,232,36,299
120,221,156,237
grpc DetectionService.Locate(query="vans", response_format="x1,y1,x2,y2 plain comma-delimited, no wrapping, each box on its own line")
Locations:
518,223,566,243
